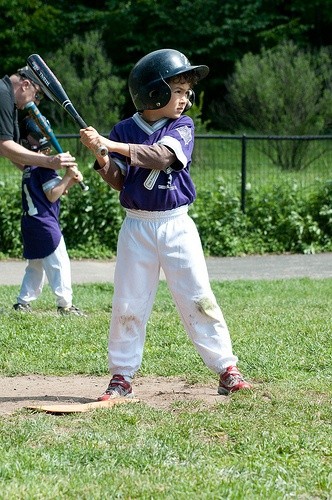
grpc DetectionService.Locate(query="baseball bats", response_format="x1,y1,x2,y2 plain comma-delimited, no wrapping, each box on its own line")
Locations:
26,54,109,158
24,101,90,192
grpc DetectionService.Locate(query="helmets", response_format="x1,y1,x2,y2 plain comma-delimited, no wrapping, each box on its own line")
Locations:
128,49,209,111
21,116,52,150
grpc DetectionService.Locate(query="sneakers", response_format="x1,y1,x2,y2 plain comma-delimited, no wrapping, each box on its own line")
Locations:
13,304,33,315
97,374,132,401
57,305,87,317
218,365,253,395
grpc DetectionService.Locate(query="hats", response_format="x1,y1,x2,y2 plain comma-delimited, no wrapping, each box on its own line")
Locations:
17,66,54,101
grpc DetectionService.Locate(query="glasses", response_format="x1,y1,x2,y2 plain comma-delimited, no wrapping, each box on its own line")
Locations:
28,78,45,99
